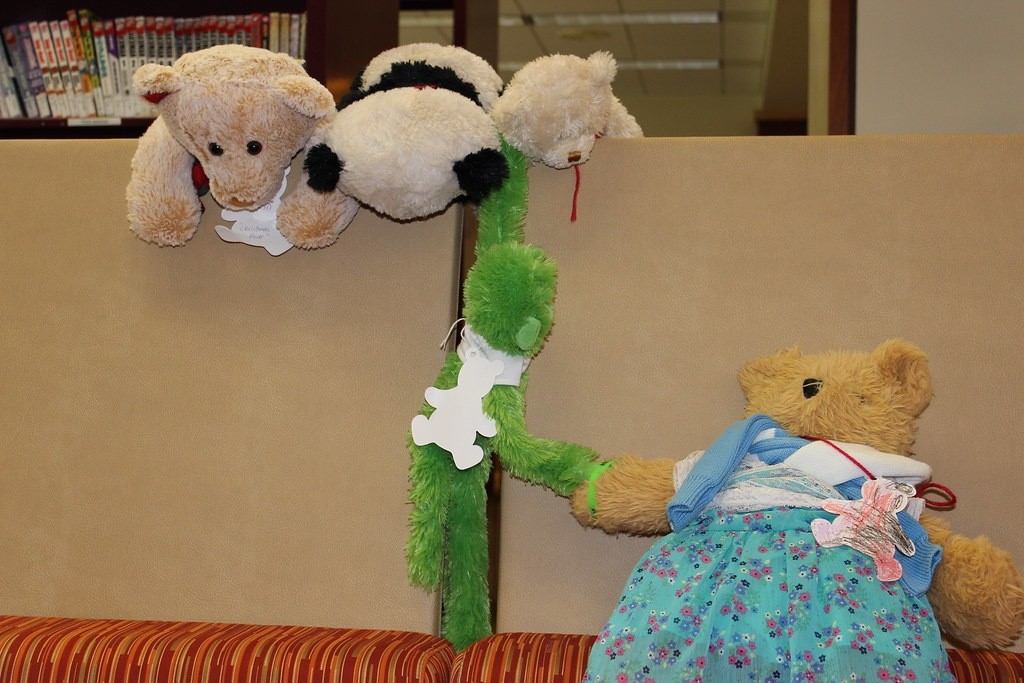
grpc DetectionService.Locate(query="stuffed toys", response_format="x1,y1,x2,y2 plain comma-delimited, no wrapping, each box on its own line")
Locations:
127,44,361,250
570,337,1024,683
401,140,599,653
304,43,511,222
493,51,643,169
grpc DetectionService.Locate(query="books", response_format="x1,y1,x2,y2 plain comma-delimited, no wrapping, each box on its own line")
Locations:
0,10,308,120
